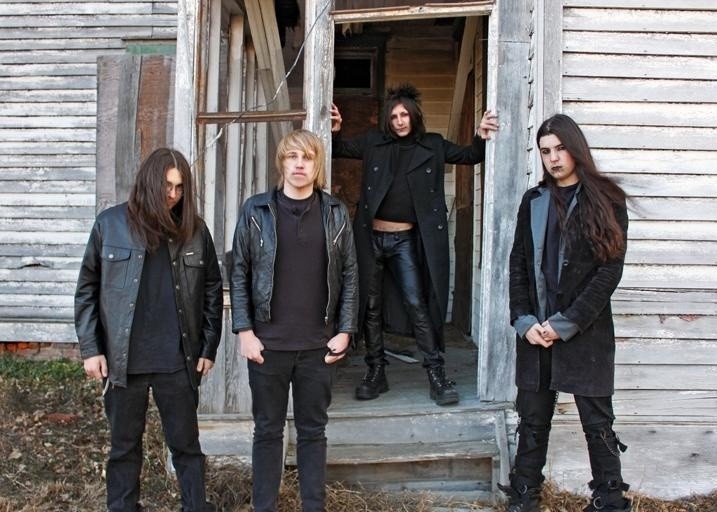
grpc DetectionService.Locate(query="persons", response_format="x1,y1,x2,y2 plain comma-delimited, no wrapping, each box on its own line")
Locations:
329,90,499,408
74,147,223,511
227,128,360,512
498,112,630,512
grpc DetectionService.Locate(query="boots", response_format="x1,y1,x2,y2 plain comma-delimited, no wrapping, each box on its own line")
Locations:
354,363,390,401
583,474,631,512
498,474,543,512
428,365,459,405
